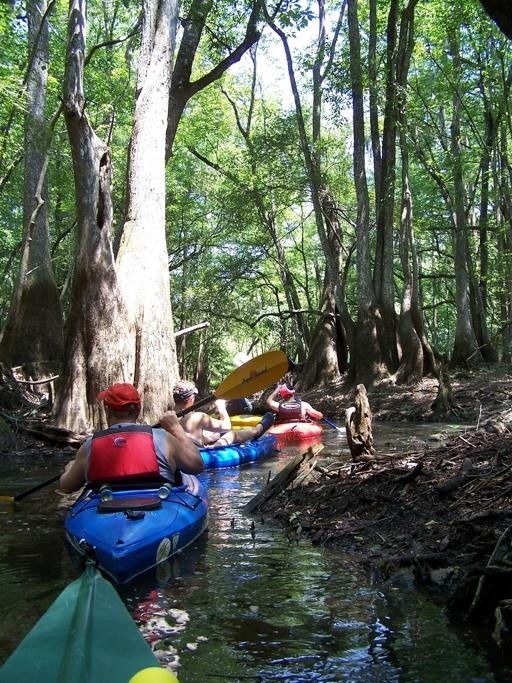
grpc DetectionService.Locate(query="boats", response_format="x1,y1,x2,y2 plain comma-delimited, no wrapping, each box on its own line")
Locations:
62,467,210,590
149,424,276,472
229,411,327,448
1,558,179,681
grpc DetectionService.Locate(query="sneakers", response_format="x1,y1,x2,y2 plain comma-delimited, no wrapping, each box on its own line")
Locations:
253,412,275,438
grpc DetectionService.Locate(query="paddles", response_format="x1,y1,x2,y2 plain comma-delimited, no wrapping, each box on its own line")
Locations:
0,351,289,536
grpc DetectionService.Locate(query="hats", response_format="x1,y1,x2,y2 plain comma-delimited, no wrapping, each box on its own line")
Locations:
280,383,294,400
98,384,141,413
173,381,198,399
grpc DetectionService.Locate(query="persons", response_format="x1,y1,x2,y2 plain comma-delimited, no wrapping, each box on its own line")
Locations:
173,379,275,450
266,381,325,422
227,399,252,415
60,383,203,495
